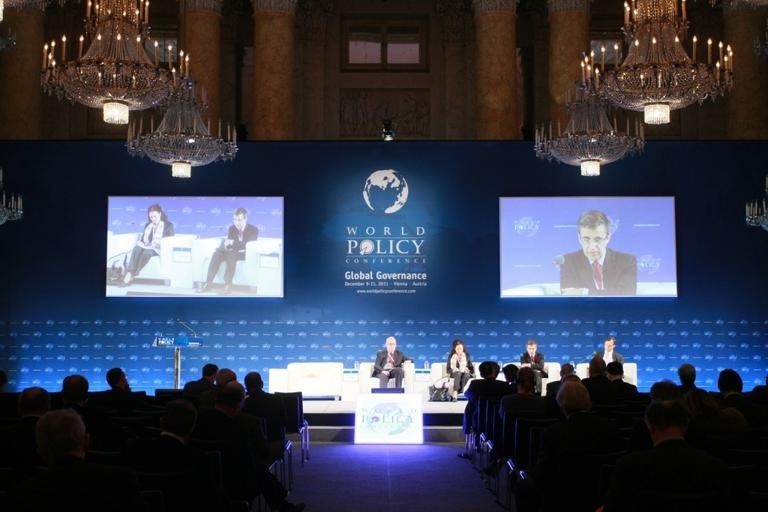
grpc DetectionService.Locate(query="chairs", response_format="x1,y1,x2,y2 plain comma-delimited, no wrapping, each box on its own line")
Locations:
1,390,313,512
460,399,761,511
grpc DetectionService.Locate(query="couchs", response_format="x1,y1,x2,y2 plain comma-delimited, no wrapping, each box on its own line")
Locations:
358,361,416,396
501,361,561,398
286,361,342,398
573,363,638,386
430,361,484,397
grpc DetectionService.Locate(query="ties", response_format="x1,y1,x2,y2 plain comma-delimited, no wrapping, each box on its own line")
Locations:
531,354,534,362
389,352,394,368
239,230,243,241
596,263,604,288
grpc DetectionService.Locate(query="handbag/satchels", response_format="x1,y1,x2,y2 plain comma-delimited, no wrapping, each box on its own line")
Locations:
429,383,450,402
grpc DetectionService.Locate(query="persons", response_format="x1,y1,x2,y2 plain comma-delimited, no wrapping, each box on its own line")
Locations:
371,335,413,389
195,207,261,296
557,210,639,297
591,334,627,382
518,339,548,378
445,338,477,403
112,205,174,287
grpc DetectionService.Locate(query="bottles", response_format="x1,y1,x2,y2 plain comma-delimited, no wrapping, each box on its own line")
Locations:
424,362,428,369
498,362,501,371
569,361,573,370
353,361,359,369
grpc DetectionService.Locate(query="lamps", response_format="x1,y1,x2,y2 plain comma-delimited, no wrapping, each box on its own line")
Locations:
575,0,741,126
533,76,648,178
36,0,191,126
124,76,240,178
